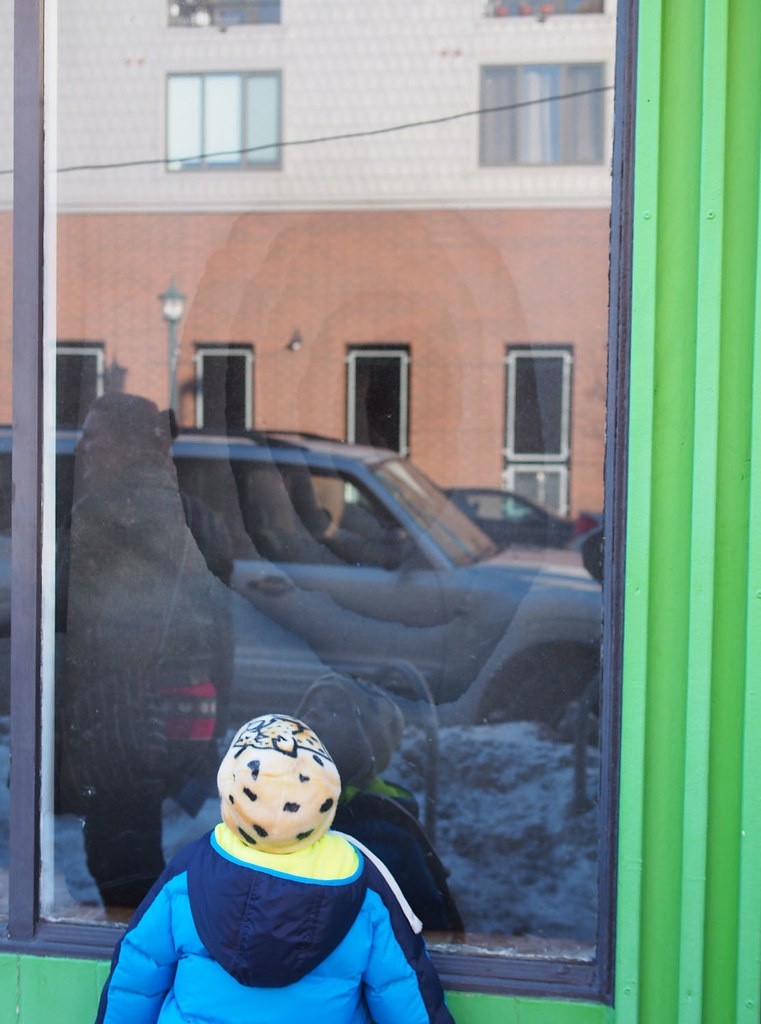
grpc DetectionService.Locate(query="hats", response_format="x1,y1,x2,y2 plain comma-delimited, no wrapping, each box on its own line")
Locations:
291,674,403,778
216,714,341,854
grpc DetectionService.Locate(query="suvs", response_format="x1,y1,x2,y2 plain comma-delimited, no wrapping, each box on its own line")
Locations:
0,425,606,740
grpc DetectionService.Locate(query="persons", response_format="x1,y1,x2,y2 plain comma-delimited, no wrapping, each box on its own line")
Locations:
62,393,211,911
93,713,459,1024
289,668,459,934
